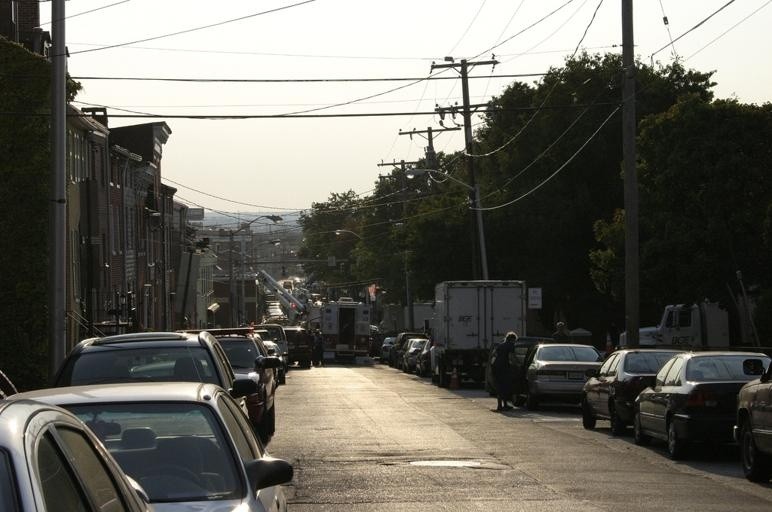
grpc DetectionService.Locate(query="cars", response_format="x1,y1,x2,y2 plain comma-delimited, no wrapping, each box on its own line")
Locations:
253,267,370,368
370,325,431,378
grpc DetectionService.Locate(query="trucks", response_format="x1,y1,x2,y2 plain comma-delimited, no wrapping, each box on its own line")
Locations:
617,300,731,353
423,277,529,394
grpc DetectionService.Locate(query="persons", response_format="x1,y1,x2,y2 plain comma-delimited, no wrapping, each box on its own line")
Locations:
552,320,569,342
491,331,519,410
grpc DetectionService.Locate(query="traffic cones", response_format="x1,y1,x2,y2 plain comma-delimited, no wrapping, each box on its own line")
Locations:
449,366,460,390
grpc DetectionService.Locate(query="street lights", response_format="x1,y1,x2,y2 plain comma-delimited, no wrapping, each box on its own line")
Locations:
336,228,360,240
241,238,280,324
228,214,283,329
405,167,491,279
